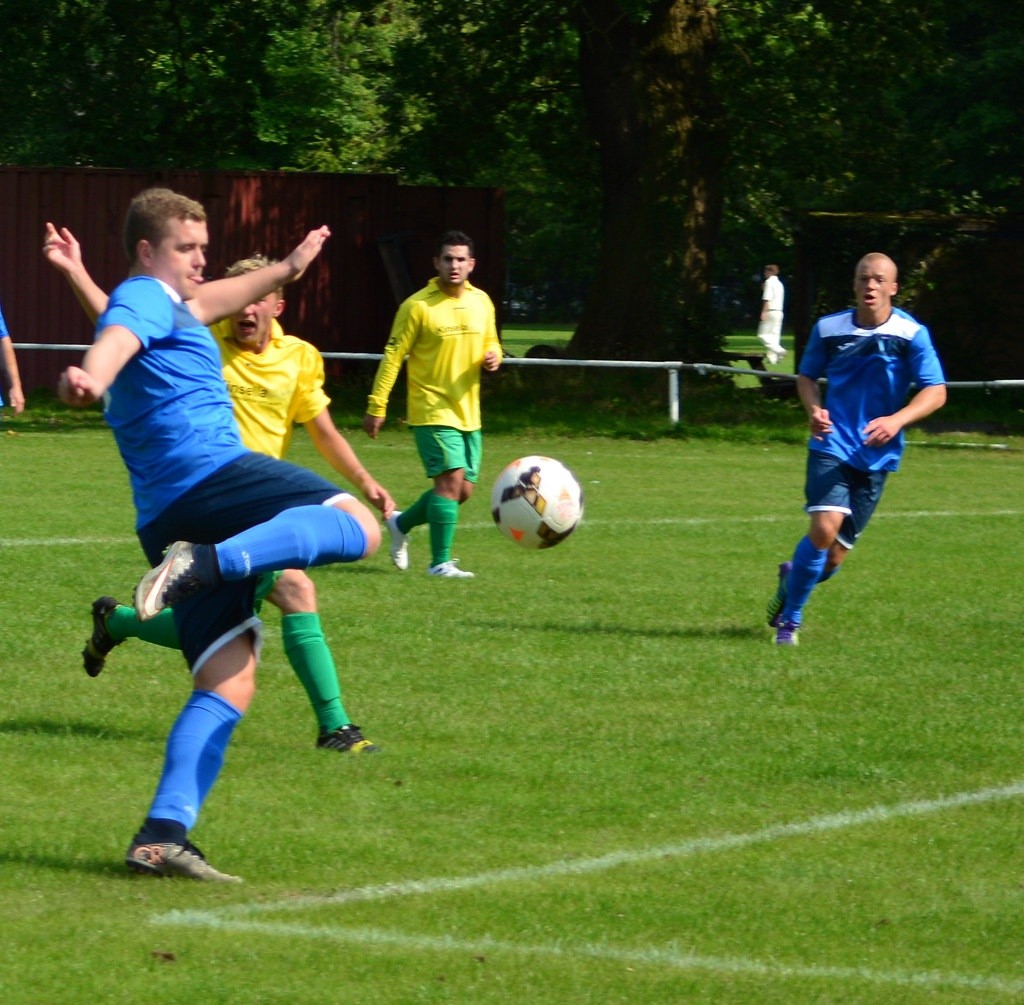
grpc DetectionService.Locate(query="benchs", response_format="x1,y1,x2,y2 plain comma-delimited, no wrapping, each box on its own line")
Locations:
722,351,770,385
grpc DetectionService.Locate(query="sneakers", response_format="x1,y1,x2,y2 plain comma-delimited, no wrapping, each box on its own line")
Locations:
766,560,792,626
132,542,199,622
315,723,378,754
776,617,800,647
429,557,475,578
386,512,408,569
80,596,121,677
124,840,241,882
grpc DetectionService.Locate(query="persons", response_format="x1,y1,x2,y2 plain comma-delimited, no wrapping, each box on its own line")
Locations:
757,265,787,364
54,188,381,884
364,229,503,578
42,223,396,755
765,253,947,645
0,308,25,417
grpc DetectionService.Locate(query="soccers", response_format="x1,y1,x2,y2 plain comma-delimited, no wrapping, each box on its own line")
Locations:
494,453,585,551
